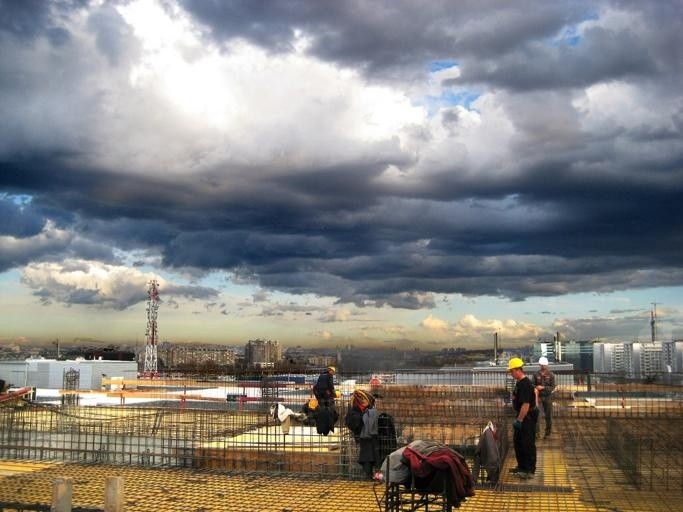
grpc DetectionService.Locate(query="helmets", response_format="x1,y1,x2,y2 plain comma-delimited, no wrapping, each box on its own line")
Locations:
328,365,337,373
335,390,341,399
508,358,524,370
537,356,550,365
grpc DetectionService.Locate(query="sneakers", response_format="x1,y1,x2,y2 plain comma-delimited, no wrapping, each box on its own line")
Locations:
536,432,551,440
510,466,534,480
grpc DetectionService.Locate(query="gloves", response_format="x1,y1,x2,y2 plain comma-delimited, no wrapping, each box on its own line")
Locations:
512,420,523,428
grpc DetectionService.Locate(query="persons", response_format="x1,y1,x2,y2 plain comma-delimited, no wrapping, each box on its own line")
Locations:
507,357,539,479
532,357,555,439
313,366,336,408
